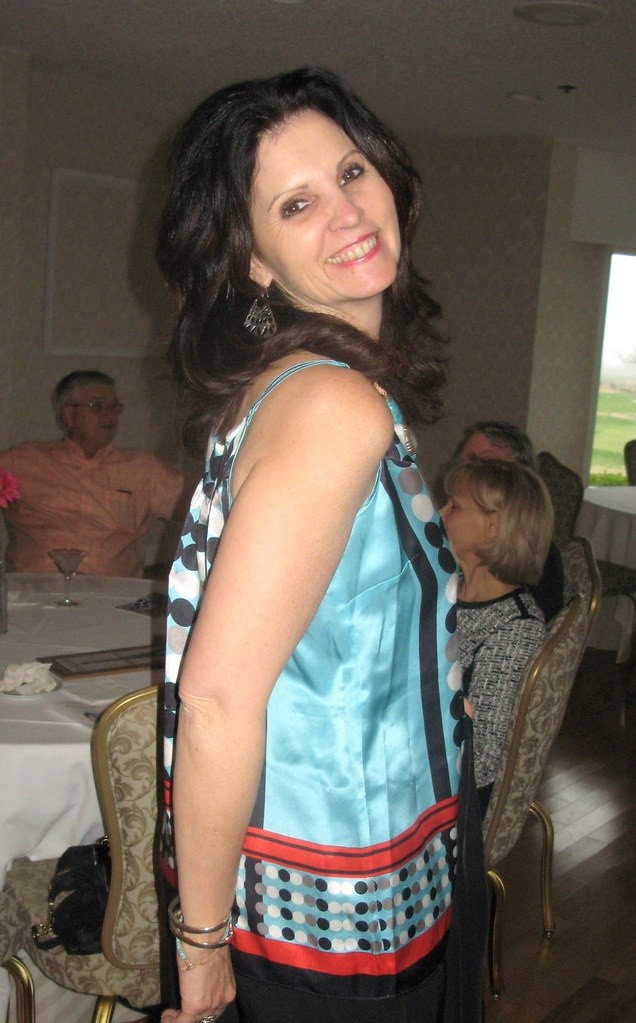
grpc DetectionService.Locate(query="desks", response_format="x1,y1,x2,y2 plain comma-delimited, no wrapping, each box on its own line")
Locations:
568,484,636,596
0,571,168,704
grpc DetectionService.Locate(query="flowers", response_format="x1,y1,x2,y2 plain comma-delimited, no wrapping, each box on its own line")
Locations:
0,465,21,509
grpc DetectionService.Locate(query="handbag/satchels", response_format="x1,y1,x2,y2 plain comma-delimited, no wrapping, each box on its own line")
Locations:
31,844,111,955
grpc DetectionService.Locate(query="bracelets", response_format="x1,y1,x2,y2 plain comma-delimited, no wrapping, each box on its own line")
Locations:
168,894,234,972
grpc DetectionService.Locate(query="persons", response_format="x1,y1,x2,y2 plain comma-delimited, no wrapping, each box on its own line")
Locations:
152,69,476,1023
0,369,187,576
437,420,565,819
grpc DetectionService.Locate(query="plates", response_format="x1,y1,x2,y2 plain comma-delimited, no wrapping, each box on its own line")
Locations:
0,669,62,701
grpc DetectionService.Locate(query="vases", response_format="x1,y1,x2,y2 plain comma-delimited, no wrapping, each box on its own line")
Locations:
0,509,10,573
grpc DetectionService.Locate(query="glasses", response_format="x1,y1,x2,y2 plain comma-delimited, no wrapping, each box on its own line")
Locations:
68,401,125,414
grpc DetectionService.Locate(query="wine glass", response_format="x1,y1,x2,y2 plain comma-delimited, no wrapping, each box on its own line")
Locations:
47,548,89,607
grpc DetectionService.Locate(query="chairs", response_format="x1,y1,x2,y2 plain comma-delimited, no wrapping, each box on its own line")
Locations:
0,683,164,1023
557,535,601,625
478,592,591,1002
534,451,583,551
